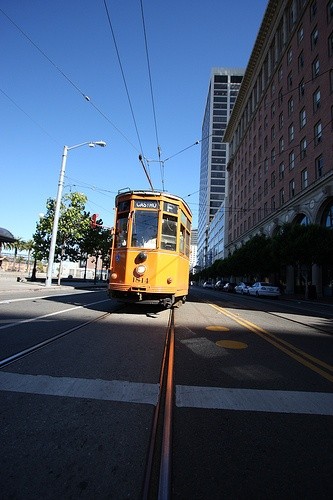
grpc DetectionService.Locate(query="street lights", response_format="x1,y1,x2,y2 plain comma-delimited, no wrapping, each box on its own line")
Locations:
45,140,107,287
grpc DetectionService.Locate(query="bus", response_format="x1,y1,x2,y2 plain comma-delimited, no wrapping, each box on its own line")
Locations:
107,186,192,309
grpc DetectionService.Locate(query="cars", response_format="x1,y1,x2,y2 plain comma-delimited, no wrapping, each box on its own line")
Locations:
234,282,255,294
203,282,214,290
214,280,229,290
223,283,237,292
246,282,281,298
